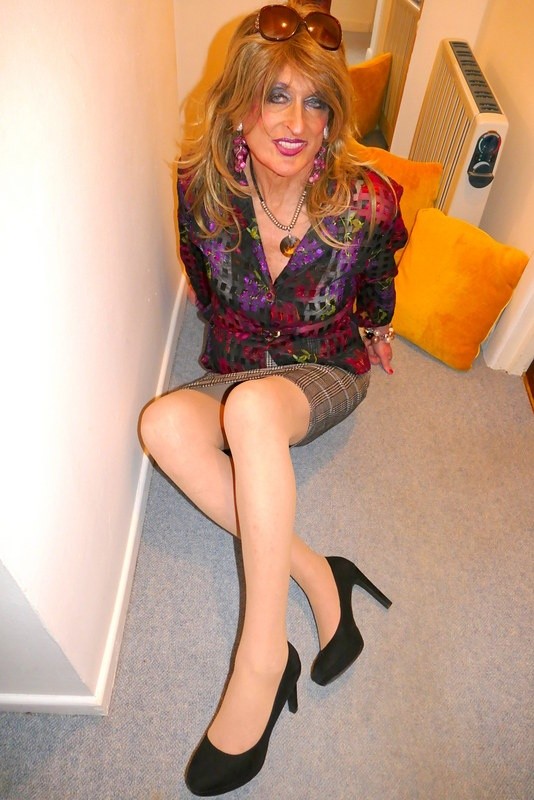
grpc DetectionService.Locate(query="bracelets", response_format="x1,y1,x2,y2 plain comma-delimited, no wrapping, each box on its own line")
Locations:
364,327,395,344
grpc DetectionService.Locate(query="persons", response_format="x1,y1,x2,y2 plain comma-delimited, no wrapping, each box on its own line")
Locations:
138,6,408,797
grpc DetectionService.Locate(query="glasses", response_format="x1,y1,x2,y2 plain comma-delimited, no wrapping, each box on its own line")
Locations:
246,4,343,52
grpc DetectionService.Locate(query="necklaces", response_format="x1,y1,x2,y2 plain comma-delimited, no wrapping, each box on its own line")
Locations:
249,153,314,259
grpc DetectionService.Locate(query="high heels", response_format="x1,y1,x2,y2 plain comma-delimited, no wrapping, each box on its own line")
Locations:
311,556,392,687
184,640,301,797
